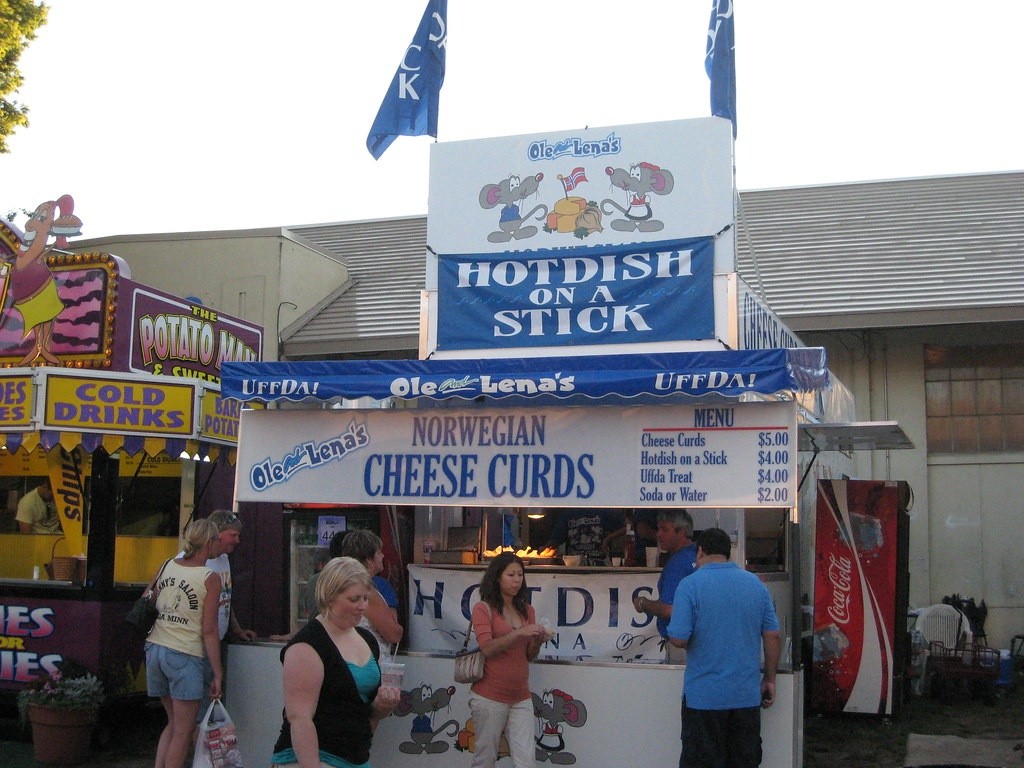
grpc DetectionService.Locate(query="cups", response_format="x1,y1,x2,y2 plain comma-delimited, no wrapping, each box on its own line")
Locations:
379,662,405,692
645,547,658,568
612,557,621,567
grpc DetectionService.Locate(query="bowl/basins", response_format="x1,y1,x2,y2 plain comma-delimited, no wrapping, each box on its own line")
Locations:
562,555,580,566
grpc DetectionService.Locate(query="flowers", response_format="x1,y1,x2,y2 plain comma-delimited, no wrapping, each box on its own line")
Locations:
18,658,104,725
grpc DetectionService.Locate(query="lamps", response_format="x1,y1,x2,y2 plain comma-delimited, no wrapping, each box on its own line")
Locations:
527,507,545,519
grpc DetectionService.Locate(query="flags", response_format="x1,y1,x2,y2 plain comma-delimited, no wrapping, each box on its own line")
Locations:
706,0,737,139
367,0,447,161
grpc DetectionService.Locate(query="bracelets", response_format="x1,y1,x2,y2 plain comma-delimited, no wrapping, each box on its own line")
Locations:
639,597,646,610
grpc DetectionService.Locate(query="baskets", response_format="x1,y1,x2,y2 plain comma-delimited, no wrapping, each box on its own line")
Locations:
51,537,78,581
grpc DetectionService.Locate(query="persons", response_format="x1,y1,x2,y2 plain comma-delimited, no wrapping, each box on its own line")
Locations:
305,531,404,661
668,528,782,768
632,508,658,568
143,518,222,768
549,508,627,566
634,509,699,665
193,511,256,747
469,552,549,768
268,557,400,768
15,478,63,533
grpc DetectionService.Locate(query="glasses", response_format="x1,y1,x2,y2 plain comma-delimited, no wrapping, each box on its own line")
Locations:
47,504,51,517
218,514,237,528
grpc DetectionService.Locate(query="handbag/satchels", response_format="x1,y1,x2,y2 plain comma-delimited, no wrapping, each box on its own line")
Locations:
191,699,243,768
124,597,158,637
453,600,492,684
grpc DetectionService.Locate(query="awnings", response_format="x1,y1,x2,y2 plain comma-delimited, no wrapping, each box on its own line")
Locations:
797,420,916,492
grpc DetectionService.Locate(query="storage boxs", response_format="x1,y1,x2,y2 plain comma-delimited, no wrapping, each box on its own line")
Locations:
971,649,1014,685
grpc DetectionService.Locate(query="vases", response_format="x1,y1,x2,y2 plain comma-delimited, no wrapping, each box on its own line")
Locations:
27,703,92,765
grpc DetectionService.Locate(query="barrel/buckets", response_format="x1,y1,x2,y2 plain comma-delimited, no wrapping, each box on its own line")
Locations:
52,556,78,582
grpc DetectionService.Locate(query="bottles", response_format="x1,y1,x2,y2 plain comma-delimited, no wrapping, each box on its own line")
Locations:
622,530,637,567
